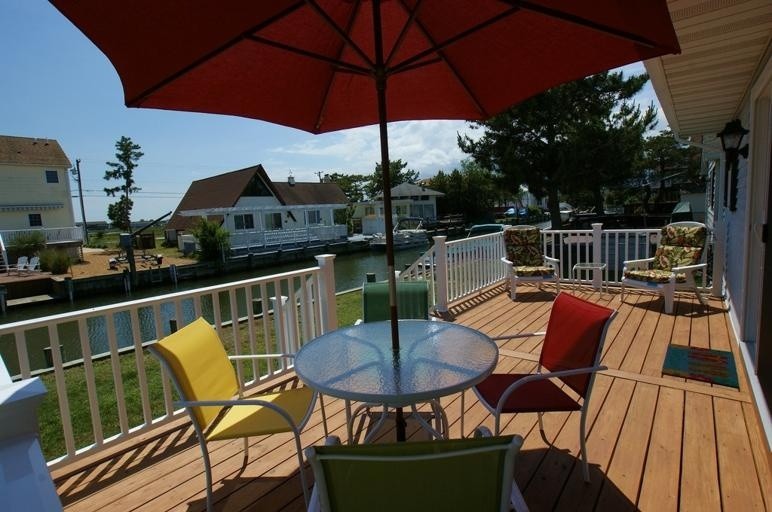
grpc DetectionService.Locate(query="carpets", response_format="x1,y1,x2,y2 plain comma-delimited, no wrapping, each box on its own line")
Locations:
661,342,741,389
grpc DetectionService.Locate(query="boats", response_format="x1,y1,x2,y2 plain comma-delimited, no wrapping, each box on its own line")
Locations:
368,217,429,248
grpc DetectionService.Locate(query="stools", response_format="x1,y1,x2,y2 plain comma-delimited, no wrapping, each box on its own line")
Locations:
573,263,609,298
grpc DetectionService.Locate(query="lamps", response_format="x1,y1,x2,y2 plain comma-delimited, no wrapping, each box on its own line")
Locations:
715,118,750,150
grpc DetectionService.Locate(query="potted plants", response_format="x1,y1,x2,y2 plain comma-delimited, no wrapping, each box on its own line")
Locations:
37,248,56,270
45,250,72,275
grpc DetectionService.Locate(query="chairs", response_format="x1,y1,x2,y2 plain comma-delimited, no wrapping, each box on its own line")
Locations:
18,257,40,275
146,317,329,511
8,256,28,277
470,290,619,483
620,221,708,314
362,281,429,322
305,426,530,512
501,225,561,300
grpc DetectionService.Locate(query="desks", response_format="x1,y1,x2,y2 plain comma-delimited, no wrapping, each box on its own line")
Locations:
294,320,500,444
